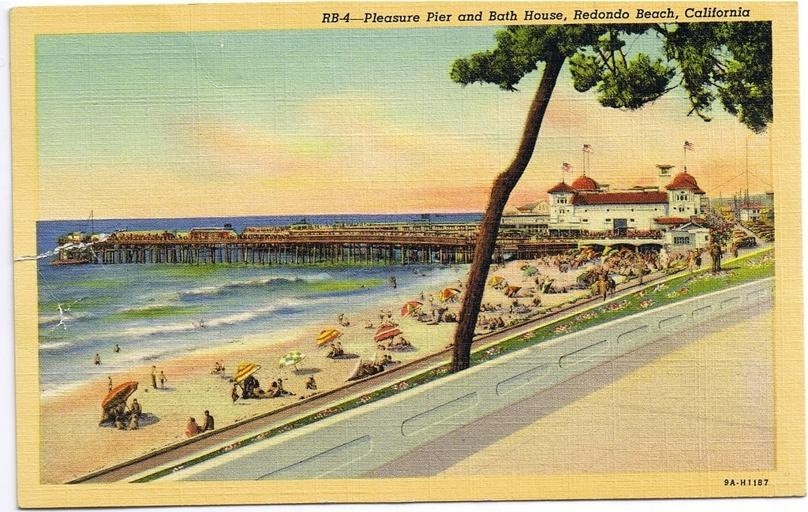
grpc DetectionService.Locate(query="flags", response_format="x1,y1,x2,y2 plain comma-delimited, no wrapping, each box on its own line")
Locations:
683,141,695,153
563,163,573,173
583,144,593,153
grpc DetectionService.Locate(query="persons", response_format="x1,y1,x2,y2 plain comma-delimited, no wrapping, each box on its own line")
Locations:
93,238,725,439
